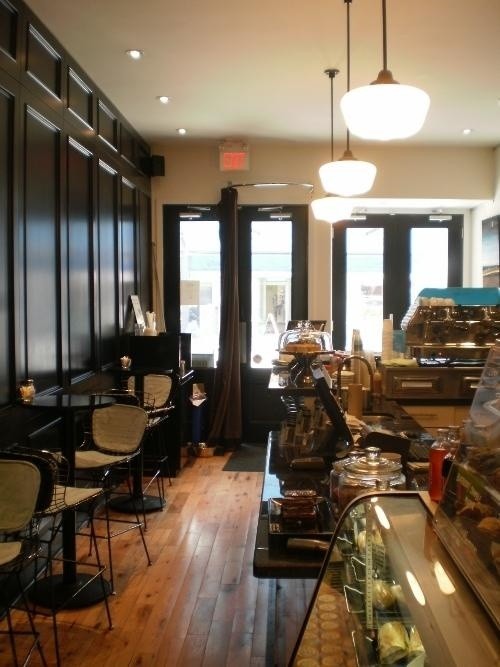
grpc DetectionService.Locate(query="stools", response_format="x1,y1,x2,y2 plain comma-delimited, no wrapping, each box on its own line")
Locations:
49,406,155,595
80,389,161,535
125,375,173,501
2,457,45,666
2,444,117,667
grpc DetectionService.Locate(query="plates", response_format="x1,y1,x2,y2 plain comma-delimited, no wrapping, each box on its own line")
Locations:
331,370,355,388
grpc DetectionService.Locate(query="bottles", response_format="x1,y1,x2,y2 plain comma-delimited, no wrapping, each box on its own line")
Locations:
427,423,464,504
326,445,408,527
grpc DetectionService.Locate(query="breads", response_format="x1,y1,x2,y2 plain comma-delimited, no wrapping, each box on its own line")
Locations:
357,530,427,667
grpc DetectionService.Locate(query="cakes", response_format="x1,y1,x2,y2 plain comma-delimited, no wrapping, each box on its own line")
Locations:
286,339,321,352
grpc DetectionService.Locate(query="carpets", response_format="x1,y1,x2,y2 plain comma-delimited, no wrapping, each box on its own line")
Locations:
222,443,266,472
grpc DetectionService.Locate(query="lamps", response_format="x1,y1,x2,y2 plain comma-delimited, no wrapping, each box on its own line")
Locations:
309,0,432,225
